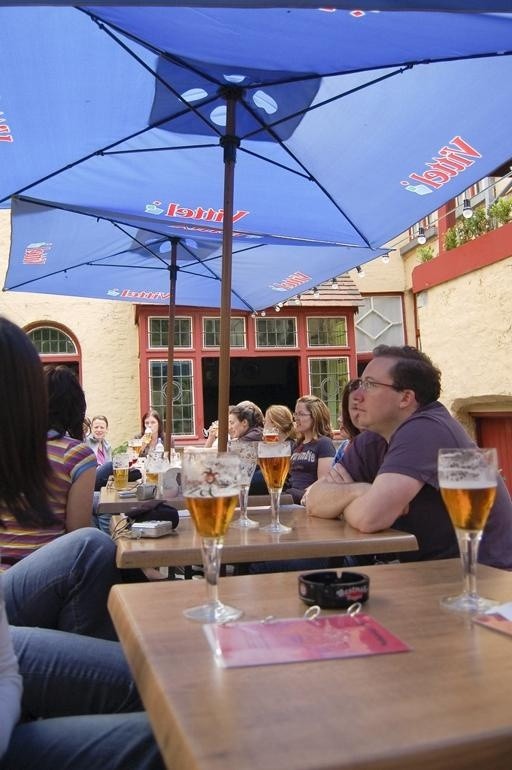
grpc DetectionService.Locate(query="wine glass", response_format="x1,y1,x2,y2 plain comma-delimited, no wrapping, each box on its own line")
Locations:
202,422,218,437
180,448,244,624
256,440,293,534
145,466,159,485
438,447,500,613
228,440,258,530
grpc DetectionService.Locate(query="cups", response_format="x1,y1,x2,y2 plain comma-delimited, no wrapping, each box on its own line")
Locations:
263,427,279,443
113,453,129,491
128,439,142,459
144,428,151,444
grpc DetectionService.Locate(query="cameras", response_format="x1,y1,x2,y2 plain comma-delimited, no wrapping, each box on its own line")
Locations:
137,484,158,501
130,519,173,538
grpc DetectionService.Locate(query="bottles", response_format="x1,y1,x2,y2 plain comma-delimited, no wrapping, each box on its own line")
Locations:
155,438,165,460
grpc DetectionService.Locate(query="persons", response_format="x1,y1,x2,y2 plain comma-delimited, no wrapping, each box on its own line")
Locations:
0,315,169,768
0,525,120,637
303,342,511,573
204,393,333,508
333,376,368,470
0,366,100,571
84,416,116,491
131,407,168,460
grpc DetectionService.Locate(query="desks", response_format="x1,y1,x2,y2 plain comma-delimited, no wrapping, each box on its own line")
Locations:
108,556,512,770
98,484,293,515
111,503,422,582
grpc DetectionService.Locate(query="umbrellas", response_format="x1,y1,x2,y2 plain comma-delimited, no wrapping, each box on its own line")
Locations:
1,0,510,455
2,194,398,453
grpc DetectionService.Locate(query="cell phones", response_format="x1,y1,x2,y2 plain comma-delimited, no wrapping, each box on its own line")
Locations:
120,493,136,498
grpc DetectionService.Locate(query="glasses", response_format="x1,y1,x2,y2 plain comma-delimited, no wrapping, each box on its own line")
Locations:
293,411,311,419
354,378,394,394
